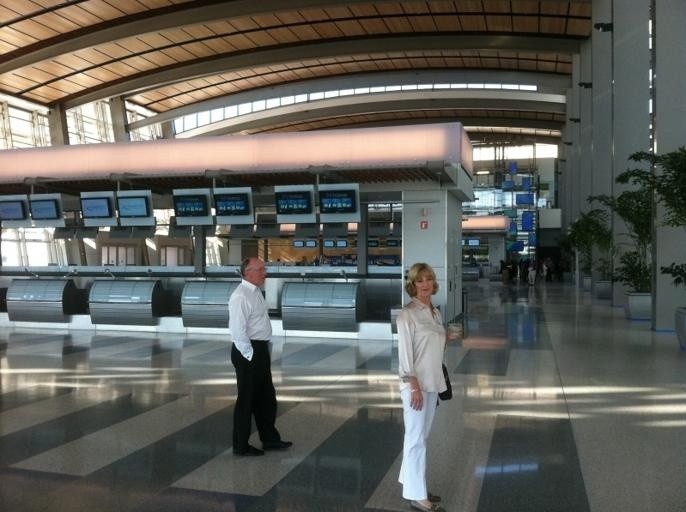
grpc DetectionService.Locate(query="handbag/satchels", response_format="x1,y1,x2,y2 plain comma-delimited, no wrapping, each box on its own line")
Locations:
437,362,452,400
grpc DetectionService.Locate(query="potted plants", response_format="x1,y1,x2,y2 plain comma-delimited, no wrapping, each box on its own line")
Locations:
554,144,685,350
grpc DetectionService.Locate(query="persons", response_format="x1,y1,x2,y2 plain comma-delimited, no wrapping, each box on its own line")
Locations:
497,257,565,286
228,255,292,456
395,263,448,512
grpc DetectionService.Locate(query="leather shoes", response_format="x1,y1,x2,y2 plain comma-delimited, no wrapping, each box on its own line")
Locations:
428,492,441,502
263,440,292,450
233,445,264,457
410,500,446,512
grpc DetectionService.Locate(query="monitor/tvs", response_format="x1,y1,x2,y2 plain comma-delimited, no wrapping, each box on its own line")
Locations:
80,197,112,218
173,194,209,218
117,196,149,218
213,193,250,216
318,190,356,213
275,191,312,214
0,200,26,220
29,199,59,220
293,238,480,248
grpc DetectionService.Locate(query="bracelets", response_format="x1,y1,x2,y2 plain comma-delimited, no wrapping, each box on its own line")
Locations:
410,388,422,392
